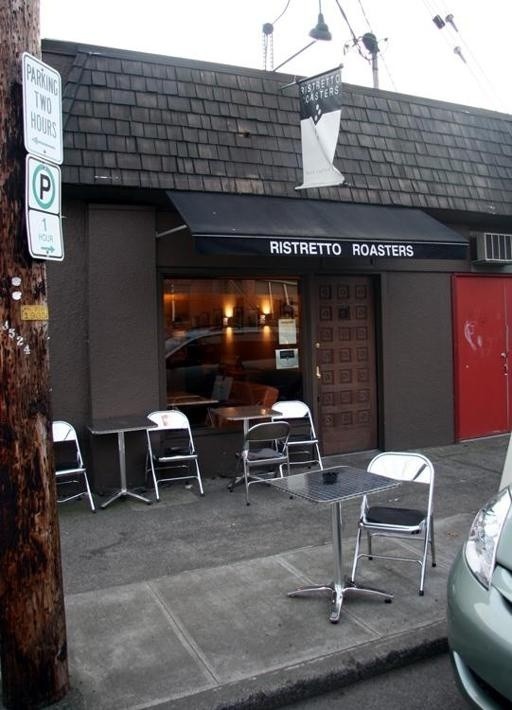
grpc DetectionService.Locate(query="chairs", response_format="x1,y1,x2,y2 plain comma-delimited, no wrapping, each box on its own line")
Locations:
145,409,205,502
51,420,96,514
271,400,323,479
230,421,293,505
352,452,436,595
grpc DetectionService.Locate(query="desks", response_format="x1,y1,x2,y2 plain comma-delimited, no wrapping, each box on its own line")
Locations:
86,413,158,509
211,406,282,488
268,465,402,625
167,392,219,429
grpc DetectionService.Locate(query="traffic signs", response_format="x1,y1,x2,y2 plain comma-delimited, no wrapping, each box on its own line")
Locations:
22,52,63,165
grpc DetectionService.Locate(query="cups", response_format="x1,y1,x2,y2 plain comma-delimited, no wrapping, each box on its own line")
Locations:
322,472,337,485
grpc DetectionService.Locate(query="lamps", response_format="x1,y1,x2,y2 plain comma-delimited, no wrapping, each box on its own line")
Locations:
263,0,331,40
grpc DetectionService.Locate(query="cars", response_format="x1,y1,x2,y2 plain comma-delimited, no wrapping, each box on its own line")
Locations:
447,482,512,710
165,326,304,393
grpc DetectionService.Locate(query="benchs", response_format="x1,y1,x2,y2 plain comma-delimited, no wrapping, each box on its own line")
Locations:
202,377,279,428
